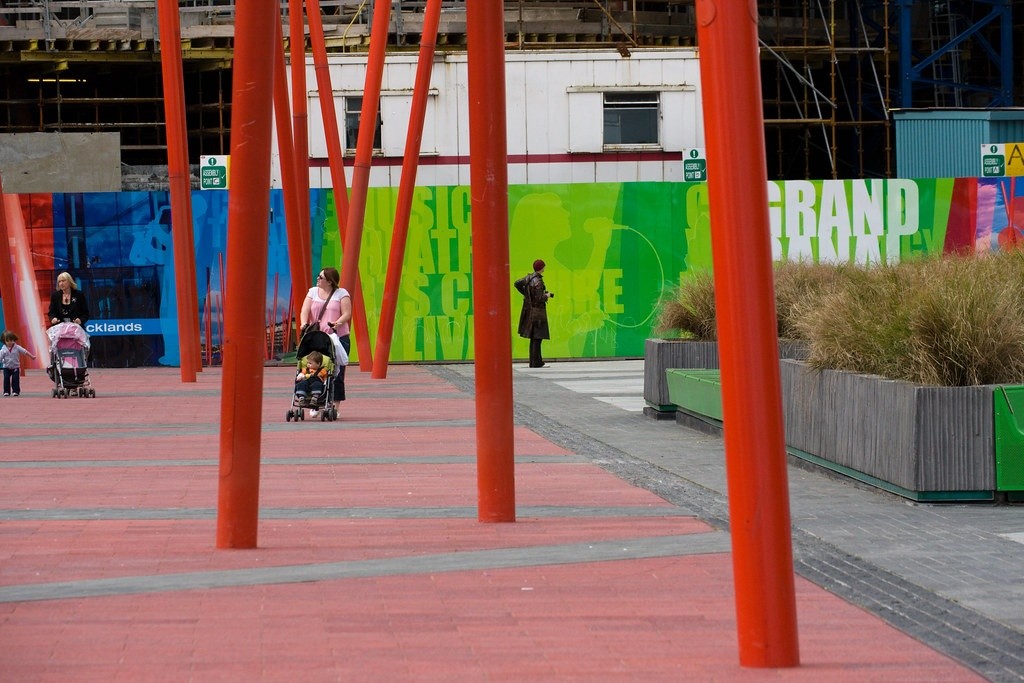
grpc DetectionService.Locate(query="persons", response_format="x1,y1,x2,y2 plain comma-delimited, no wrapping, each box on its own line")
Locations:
514,259,550,368
0,330,37,397
300,268,351,418
48,271,89,395
297,351,328,407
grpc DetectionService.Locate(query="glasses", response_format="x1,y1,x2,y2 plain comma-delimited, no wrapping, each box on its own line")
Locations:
318,274,327,280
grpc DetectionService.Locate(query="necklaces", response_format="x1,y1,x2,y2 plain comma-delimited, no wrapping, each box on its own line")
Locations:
64,294,69,303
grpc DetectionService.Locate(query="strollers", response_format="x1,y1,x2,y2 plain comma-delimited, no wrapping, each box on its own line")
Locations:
44,317,96,399
286,321,337,422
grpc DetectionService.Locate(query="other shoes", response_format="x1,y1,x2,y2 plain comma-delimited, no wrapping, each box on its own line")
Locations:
4,393,9,396
310,400,317,406
13,393,18,396
299,398,306,406
310,409,318,417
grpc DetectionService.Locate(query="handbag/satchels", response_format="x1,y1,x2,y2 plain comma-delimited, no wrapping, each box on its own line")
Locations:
301,322,320,338
330,332,349,366
530,308,546,323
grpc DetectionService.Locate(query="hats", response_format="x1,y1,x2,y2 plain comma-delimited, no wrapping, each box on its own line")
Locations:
534,260,545,271
